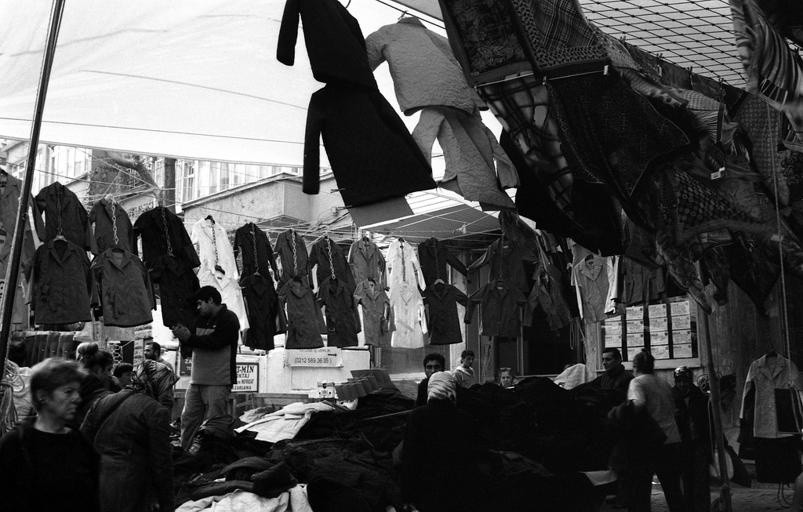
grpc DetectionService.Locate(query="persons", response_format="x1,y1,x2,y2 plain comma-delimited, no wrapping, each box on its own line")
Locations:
401,346,715,512
173,286,240,451
0,342,179,512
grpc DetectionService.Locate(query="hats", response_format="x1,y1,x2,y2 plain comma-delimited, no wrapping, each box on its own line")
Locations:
113,362,133,378
674,365,694,378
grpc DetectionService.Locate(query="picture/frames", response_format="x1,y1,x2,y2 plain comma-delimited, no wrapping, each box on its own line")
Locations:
230,363,259,393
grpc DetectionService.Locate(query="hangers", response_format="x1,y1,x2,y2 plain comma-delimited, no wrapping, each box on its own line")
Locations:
765,340,778,358
